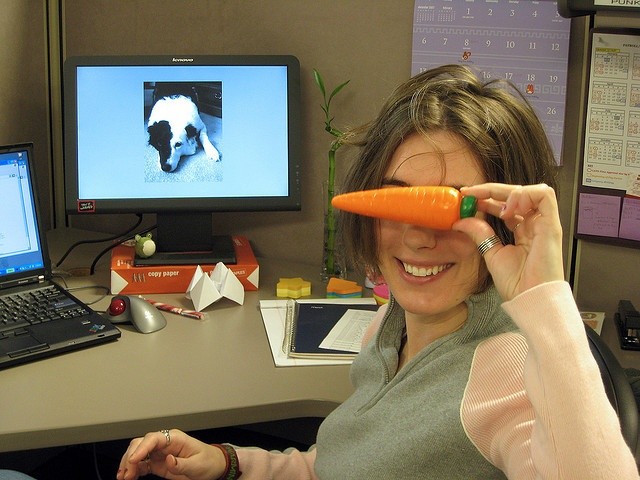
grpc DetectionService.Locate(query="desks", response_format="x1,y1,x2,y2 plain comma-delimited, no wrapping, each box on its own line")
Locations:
1,222,639,455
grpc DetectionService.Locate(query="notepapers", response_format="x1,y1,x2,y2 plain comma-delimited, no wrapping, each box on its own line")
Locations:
372,285,390,305
326,277,363,299
276,276,311,299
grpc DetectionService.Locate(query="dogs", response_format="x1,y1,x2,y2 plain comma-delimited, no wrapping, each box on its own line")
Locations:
147,82,223,173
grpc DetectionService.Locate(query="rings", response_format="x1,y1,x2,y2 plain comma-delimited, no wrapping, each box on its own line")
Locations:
144,460,152,474
511,220,524,232
160,429,170,445
477,234,501,257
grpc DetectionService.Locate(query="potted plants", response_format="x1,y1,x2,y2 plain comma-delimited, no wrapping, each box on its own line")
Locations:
309,67,353,280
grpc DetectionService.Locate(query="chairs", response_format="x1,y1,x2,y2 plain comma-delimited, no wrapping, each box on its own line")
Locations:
578,317,638,467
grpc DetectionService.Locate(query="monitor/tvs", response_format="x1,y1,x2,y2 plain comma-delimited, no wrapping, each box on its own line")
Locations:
61,53,302,267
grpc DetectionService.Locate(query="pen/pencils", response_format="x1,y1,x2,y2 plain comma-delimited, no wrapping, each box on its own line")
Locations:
143,299,204,320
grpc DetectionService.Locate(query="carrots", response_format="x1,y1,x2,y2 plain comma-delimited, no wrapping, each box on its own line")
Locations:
331,186,478,232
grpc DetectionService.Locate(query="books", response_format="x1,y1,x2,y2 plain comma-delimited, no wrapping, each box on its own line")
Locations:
282,298,379,362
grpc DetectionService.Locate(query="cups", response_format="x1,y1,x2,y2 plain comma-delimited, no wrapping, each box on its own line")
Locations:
320,181,350,285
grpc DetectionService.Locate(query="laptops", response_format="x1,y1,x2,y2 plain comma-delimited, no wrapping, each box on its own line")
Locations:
1,143,124,369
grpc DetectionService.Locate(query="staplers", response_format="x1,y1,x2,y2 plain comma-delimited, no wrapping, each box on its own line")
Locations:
614,300,640,350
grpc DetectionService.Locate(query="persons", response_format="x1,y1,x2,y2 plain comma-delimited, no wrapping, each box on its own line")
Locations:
115,66,640,480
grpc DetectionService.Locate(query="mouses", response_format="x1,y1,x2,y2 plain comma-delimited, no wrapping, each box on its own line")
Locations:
103,292,167,335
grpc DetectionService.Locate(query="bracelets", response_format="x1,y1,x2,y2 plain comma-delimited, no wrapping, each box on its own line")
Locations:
210,443,240,480
